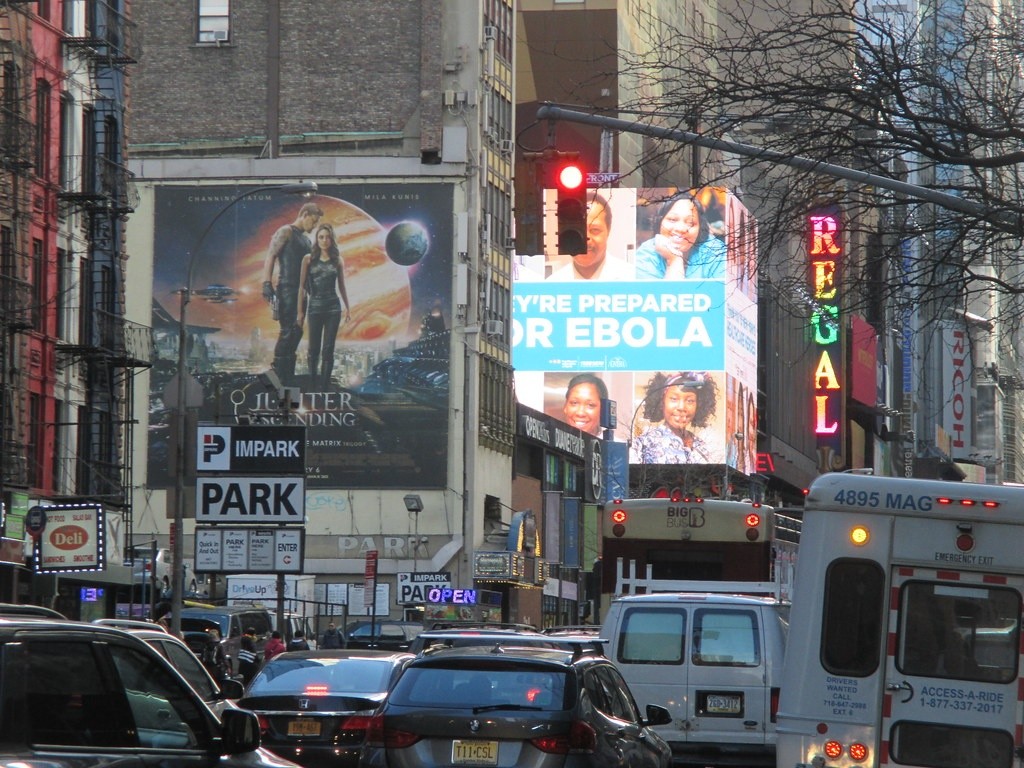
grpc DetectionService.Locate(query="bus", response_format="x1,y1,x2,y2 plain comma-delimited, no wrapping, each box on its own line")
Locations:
593,493,801,605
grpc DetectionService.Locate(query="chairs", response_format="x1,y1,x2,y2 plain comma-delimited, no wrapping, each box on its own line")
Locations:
452,684,483,704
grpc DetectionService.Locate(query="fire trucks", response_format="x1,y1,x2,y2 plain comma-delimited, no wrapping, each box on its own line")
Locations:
772,467,1023,767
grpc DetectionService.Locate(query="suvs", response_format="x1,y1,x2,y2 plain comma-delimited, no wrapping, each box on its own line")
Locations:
358,636,674,768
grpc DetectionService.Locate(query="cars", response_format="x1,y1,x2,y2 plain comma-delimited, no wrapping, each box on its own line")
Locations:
190,563,228,598
123,546,198,595
0,601,604,768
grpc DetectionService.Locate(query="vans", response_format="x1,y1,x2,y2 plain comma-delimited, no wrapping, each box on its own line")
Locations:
597,592,791,745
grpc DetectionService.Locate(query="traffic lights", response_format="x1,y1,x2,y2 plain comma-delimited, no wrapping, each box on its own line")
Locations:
551,161,593,256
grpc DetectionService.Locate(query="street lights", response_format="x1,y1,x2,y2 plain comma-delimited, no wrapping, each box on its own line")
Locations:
404,493,431,607
169,177,321,639
626,379,706,497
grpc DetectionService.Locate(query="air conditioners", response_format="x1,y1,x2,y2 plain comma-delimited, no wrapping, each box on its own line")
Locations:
487,320,503,335
214,30,229,42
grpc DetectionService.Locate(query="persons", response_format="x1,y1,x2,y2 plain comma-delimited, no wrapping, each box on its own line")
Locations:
635,190,725,280
262,202,324,382
726,198,758,302
238,627,268,686
725,375,759,476
540,192,633,279
286,630,312,651
263,631,285,665
630,370,718,464
321,623,346,649
563,373,629,445
295,222,350,397
200,629,231,685
